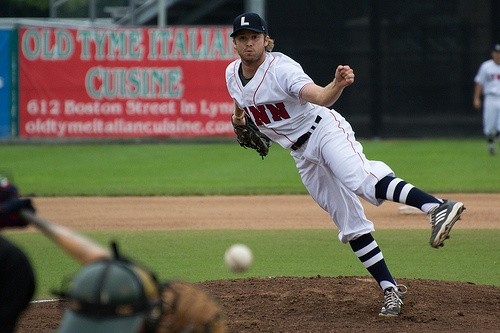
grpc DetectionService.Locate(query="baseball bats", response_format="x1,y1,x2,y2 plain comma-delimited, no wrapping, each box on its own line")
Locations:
21,211,158,288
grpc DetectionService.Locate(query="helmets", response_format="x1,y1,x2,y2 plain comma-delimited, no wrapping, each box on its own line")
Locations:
56,259,163,333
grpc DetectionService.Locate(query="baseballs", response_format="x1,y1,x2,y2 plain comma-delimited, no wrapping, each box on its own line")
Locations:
225,245,252,272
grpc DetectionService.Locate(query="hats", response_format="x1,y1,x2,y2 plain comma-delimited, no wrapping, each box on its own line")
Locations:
230,13,267,38
492,44,500,52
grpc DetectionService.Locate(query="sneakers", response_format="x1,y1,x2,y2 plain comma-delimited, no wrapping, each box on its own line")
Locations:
428,199,466,248
379,285,407,317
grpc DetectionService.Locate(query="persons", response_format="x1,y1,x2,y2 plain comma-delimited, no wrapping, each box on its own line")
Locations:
0,177,234,333
225,14,466,319
472,46,500,154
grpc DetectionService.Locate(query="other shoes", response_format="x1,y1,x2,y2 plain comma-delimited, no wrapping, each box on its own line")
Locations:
489,146,495,155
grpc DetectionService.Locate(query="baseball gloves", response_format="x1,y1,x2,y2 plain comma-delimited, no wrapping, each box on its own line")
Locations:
230,114,271,156
157,280,226,333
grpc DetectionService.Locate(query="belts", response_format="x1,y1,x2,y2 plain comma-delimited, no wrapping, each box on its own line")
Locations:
290,115,322,151
